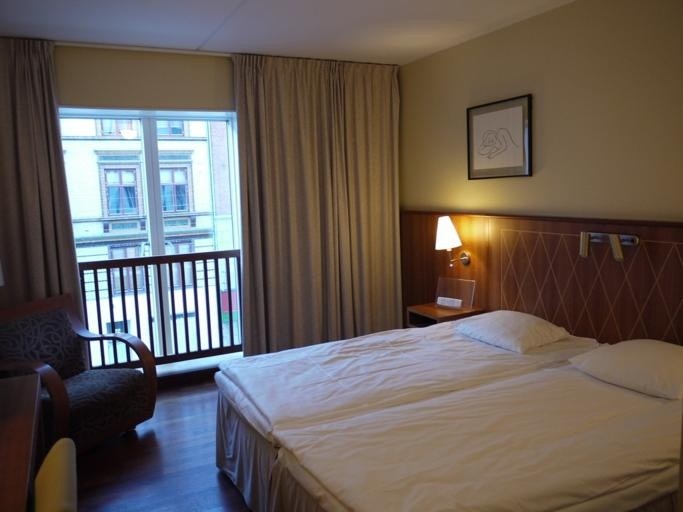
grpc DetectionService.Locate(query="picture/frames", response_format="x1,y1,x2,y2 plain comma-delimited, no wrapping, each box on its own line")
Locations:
466,94,532,180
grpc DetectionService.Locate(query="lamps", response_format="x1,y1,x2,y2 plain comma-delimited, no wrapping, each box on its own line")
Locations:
435,216,470,268
579,230,638,261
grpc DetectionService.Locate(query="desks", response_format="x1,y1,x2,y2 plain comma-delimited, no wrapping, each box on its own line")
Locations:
0,373,42,512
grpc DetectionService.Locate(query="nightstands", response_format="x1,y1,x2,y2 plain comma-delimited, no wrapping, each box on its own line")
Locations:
406,301,484,328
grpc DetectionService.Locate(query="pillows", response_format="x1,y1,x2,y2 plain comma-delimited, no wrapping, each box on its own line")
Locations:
569,339,683,400
453,310,570,353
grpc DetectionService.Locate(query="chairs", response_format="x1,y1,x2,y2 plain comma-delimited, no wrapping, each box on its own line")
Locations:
35,437,78,512
0,294,156,459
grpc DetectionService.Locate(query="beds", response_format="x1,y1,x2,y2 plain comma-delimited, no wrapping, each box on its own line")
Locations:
269,352,683,512
213,308,597,511
